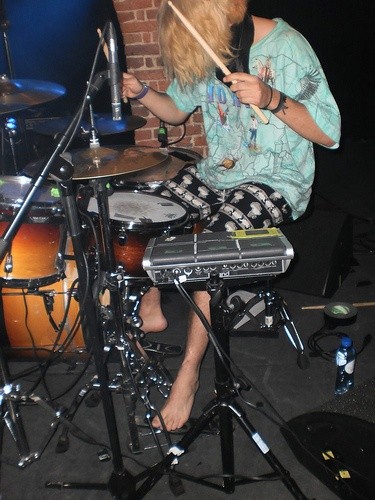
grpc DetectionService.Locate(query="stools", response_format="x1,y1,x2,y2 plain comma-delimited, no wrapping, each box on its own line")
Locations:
221,203,311,369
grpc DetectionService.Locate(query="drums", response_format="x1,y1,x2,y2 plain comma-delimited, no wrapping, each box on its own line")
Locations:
0,173,65,288
1,221,109,362
71,188,197,278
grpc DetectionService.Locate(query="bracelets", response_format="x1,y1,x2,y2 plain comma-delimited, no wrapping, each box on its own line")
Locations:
264,84,273,108
135,82,148,99
270,92,288,115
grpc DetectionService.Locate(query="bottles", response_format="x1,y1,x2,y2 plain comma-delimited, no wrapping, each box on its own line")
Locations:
334,337,356,395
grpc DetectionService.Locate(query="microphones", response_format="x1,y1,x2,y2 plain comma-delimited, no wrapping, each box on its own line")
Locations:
107,21,122,121
158,120,167,141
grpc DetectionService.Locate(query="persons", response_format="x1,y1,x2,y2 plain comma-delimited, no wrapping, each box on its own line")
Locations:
120,0,342,432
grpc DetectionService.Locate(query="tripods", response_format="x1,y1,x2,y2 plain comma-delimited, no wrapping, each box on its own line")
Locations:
0,63,306,500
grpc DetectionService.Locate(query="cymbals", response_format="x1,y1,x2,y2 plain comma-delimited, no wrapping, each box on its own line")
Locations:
111,147,206,184
22,144,170,181
1,76,65,115
33,112,147,137
